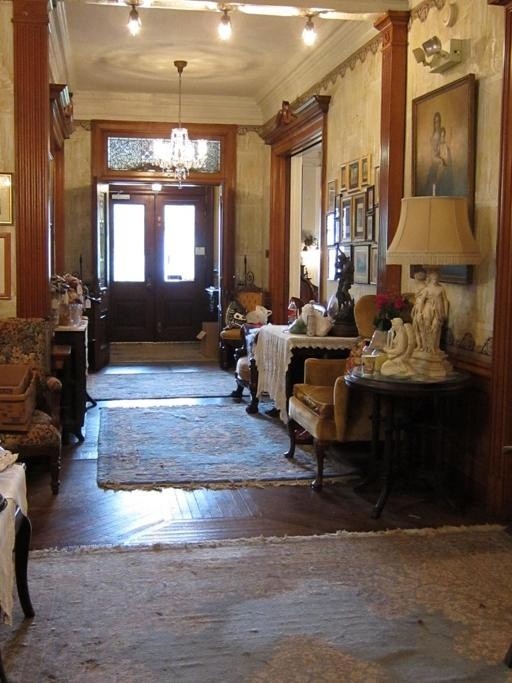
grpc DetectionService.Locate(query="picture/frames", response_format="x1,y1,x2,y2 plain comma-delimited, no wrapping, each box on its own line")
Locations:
325,151,377,286
0,232,12,298
1,171,13,222
411,70,478,288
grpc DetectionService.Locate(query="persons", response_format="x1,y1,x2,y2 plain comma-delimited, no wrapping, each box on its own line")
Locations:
334,253,355,314
421,113,442,192
379,271,451,380
443,129,454,191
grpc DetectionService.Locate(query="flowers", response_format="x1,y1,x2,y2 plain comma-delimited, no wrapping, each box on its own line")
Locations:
52,274,89,306
368,289,414,328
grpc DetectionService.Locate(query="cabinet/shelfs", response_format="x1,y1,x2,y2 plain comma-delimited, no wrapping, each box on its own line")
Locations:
52,315,97,443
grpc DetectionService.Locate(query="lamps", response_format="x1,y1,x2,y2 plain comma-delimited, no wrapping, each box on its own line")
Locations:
385,196,483,380
153,56,210,185
124,0,327,42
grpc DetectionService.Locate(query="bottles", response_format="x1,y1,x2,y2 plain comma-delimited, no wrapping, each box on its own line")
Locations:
287,300,298,324
361,340,372,354
306,300,316,336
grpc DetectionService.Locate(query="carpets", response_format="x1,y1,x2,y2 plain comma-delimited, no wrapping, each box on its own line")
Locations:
88,368,254,399
95,401,384,493
0,523,512,681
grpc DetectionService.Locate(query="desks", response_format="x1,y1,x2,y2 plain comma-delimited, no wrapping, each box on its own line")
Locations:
244,325,372,432
0,446,42,681
0,367,38,436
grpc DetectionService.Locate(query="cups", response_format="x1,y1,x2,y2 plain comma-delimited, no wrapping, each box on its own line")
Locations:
360,354,377,374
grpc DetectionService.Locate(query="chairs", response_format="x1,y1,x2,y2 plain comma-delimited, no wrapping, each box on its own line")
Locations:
220,271,269,370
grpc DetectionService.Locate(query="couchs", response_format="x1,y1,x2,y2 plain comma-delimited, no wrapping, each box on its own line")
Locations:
282,291,415,491
0,317,66,496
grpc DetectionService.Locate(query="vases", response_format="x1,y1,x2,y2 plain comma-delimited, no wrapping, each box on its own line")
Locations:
53,303,85,325
364,327,389,371
